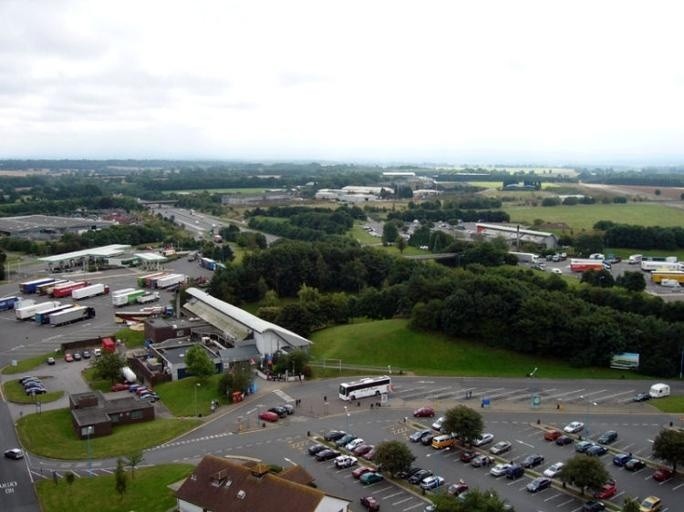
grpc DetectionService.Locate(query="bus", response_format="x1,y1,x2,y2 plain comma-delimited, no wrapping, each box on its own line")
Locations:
115,310,158,323
338,374,391,400
102,337,116,353
649,383,670,398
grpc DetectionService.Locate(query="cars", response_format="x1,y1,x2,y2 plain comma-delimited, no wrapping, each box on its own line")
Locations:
490,455,565,492
590,253,621,263
47,348,101,365
112,382,160,403
638,496,662,512
3,448,24,460
308,430,384,485
259,404,295,422
544,421,617,457
634,391,649,401
413,407,435,418
583,483,616,512
20,376,47,395
546,252,567,262
408,469,468,496
474,433,511,454
410,416,462,449
652,469,672,481
461,450,494,467
613,453,646,472
359,496,380,511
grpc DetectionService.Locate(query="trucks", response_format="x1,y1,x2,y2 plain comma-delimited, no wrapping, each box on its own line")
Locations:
201,258,217,270
137,272,184,289
641,257,684,283
661,278,680,287
141,306,174,318
112,288,145,306
0,295,95,328
215,235,222,243
629,254,642,264
571,258,611,272
136,291,160,304
19,277,110,301
